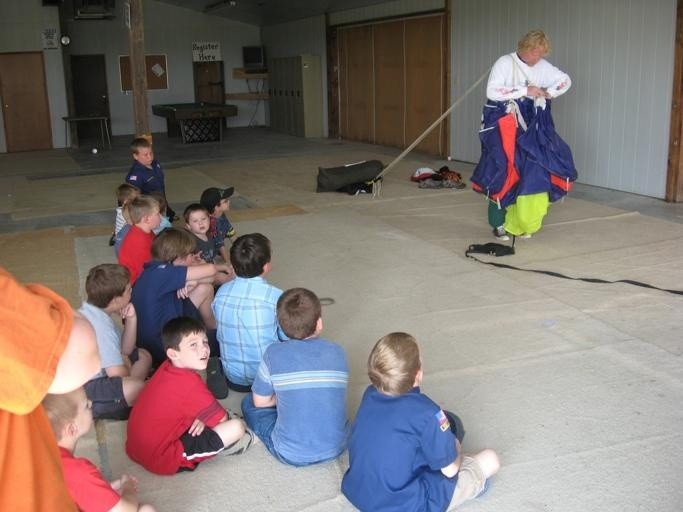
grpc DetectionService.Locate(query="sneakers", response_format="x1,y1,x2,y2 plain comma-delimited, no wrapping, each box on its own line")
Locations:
491,223,534,242
203,355,230,400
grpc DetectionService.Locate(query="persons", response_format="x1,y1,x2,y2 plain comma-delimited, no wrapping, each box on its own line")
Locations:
340,332,500,512
133,225,231,371
206,230,290,399
74,263,154,421
239,287,351,468
469,28,573,244
124,316,253,476
108,136,239,287
40,385,155,512
0,267,103,512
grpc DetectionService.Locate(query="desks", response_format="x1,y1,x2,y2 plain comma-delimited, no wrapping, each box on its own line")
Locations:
152,101,236,145
60,115,111,150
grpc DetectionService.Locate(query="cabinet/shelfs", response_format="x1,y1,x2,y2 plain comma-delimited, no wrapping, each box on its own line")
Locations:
223,68,270,101
267,55,322,139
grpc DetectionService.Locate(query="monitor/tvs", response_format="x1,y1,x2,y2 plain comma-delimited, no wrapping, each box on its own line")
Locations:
242,44,264,67
74,0,115,20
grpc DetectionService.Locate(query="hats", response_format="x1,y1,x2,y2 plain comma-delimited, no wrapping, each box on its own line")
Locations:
200,186,234,212
440,409,466,445
